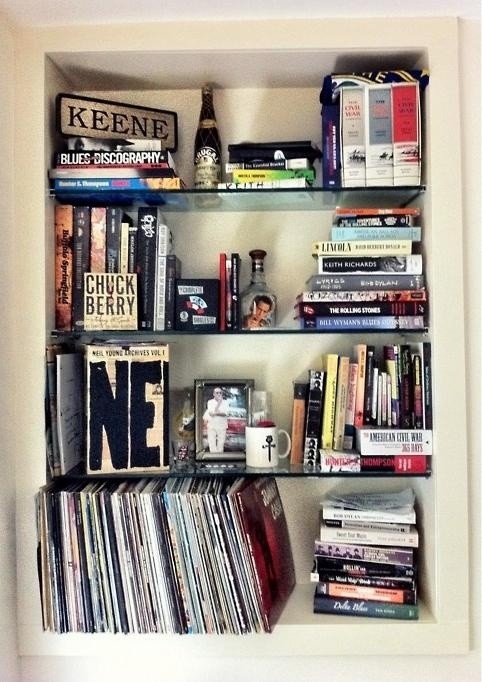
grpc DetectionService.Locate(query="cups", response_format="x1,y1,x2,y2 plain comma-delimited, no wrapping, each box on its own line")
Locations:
244,421,291,468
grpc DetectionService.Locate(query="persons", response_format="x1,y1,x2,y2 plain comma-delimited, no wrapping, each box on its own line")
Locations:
203,387,228,453
242,295,273,329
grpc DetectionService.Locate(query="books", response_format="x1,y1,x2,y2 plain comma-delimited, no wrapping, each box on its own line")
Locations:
48,338,170,474
293,208,428,331
35,474,296,634
310,489,418,621
48,138,193,211
218,140,323,189
288,342,433,474
319,68,432,188
54,204,240,333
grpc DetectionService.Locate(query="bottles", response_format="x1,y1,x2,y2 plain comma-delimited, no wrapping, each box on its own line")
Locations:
192,84,222,193
240,249,278,331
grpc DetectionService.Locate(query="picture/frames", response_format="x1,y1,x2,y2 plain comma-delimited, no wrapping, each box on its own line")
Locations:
195,380,255,460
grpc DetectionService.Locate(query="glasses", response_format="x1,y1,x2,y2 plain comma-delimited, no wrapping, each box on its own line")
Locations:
216,392,222,394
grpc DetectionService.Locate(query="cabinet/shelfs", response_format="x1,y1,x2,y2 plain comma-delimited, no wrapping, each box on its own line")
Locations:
16,15,472,657
